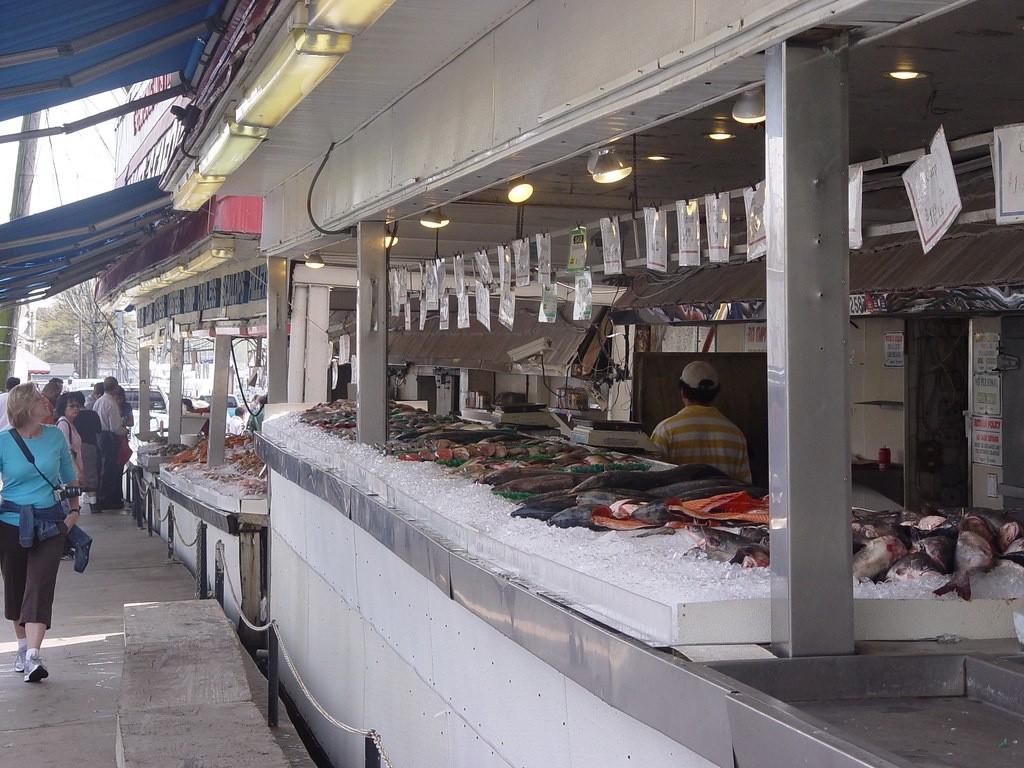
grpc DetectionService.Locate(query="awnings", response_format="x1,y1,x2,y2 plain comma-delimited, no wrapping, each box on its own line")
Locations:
0,175,185,311
0,0,238,144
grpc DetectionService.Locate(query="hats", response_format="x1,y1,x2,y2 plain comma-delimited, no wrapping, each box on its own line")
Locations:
680,360,719,389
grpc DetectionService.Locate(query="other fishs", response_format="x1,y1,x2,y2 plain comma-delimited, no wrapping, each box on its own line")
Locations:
299,395,1023,602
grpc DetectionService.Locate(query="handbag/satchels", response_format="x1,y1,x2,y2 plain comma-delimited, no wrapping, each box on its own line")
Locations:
118,434,134,465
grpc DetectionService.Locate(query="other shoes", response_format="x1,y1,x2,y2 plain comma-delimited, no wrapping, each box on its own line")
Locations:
102,501,124,509
82,492,97,505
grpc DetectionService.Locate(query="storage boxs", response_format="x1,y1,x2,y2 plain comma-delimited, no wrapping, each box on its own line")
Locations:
135,450,172,472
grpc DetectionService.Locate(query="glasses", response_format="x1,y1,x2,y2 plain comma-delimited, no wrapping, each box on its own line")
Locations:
66,403,79,408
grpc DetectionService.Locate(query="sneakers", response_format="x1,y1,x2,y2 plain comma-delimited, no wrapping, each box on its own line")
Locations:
23,651,48,682
14,647,27,672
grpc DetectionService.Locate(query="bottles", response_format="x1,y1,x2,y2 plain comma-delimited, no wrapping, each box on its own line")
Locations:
879,445,891,471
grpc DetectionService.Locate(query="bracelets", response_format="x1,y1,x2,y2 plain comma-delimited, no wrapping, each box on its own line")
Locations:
69,509,79,514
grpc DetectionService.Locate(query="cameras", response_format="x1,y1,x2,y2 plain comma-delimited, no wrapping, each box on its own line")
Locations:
53,483,82,502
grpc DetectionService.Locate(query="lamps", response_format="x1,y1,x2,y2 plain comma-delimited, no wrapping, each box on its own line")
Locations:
507,177,533,202
171,0,396,210
420,207,450,229
593,145,632,183
386,226,399,249
731,88,765,123
100,236,233,312
304,249,325,269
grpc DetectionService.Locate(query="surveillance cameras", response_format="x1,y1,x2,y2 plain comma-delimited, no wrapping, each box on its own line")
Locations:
508,337,553,363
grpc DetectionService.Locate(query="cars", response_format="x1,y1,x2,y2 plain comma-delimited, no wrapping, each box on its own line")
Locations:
197,394,240,418
62,376,169,452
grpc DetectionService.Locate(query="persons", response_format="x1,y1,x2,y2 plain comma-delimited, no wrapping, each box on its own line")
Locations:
0,377,20,429
230,395,267,435
92,376,128,510
186,406,210,414
0,381,82,682
75,391,102,514
113,385,134,504
55,392,84,561
84,382,104,410
39,378,63,424
649,361,751,483
